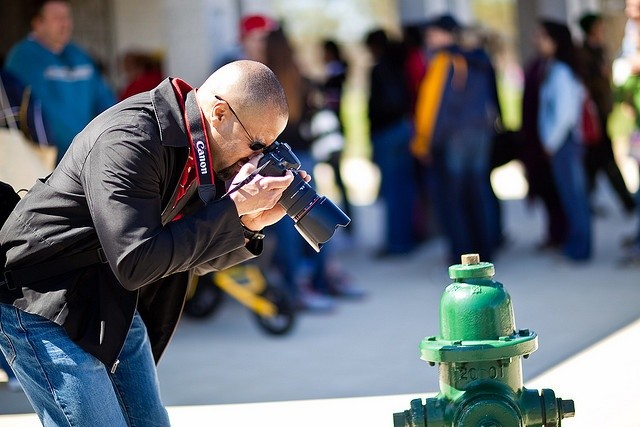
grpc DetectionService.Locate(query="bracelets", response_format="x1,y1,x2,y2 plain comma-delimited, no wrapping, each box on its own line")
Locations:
239,221,266,242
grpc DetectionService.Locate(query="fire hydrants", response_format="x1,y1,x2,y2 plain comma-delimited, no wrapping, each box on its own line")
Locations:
392,251,575,427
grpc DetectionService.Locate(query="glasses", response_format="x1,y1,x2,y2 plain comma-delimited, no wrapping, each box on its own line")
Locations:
215,94,267,151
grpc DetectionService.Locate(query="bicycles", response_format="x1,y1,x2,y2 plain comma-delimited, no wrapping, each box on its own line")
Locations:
187,255,292,335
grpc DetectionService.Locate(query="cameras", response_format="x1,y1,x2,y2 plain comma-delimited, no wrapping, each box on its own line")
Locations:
256,140,351,254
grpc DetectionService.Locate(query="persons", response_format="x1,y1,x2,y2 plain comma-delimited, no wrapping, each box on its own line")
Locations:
208,13,277,69
410,12,505,266
117,48,166,101
5,1,116,167
262,28,362,311
365,30,416,256
520,52,568,248
580,12,640,212
0,60,289,426
302,36,350,233
401,21,432,123
538,19,590,261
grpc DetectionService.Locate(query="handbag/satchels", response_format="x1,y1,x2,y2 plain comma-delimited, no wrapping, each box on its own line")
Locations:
584,97,600,142
